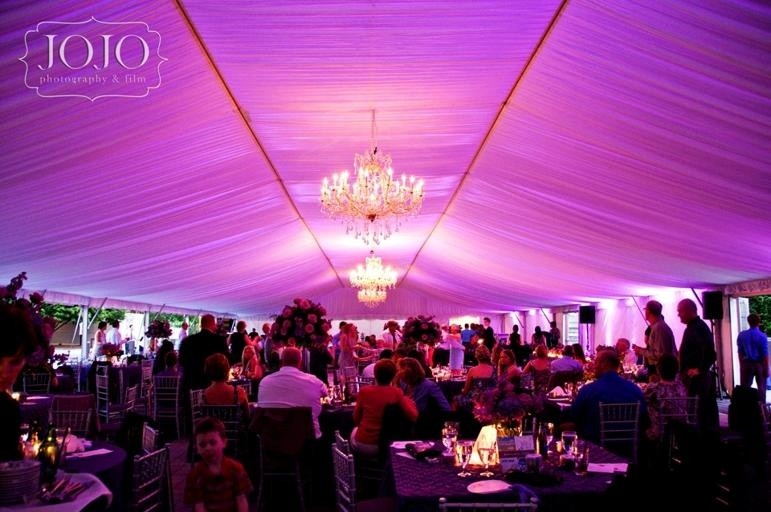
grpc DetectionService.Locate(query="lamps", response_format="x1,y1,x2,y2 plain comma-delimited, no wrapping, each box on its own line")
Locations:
320,111,426,247
346,250,399,312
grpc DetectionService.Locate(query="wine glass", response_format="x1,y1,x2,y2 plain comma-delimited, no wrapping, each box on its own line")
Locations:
334,385,341,403
442,421,495,477
538,421,590,476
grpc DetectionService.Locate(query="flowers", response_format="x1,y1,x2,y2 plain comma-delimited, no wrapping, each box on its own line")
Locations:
267,296,333,350
97,343,121,356
402,313,444,348
144,319,172,337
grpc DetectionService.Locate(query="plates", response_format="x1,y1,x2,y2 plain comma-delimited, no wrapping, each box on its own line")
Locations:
466,480,510,494
0,461,41,505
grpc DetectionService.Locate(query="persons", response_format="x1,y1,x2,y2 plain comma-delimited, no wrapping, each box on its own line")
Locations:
397,356,451,415
92,321,107,346
184,415,255,512
460,317,521,393
0,302,38,463
675,299,716,430
257,346,330,441
151,313,280,416
736,313,771,404
349,359,421,454
106,319,129,351
331,319,433,377
523,297,687,461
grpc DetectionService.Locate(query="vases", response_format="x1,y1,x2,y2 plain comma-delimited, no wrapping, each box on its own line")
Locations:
150,338,158,351
106,355,112,363
416,341,429,361
301,349,310,373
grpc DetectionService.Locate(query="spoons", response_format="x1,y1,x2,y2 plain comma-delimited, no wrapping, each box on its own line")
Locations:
42,476,95,503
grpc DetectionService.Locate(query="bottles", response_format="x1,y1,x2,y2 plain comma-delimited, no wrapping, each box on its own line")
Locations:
343,377,352,402
39,408,58,479
